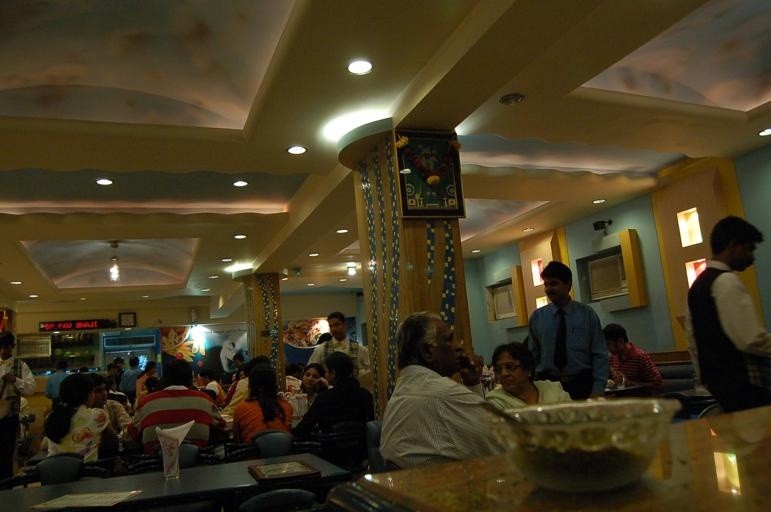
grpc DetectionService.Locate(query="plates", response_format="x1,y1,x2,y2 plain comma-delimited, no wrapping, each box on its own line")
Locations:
284,337,321,350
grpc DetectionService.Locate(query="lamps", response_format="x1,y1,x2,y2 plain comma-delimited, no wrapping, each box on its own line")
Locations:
591,218,614,235
111,241,121,261
347,265,356,277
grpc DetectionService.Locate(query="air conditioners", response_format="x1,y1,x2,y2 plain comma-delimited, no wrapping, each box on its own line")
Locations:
588,254,631,304
493,283,518,320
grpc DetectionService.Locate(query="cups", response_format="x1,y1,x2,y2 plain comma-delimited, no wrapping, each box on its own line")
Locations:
161,446,182,478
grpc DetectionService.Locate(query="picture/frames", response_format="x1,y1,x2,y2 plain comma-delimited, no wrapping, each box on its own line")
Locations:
392,125,467,223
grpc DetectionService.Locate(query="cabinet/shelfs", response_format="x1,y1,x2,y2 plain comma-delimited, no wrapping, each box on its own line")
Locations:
53,334,95,366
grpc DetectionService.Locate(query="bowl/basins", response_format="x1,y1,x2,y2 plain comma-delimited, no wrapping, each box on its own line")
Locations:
492,396,686,489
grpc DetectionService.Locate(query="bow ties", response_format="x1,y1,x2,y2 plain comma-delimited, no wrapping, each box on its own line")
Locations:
0,360,10,366
332,343,345,349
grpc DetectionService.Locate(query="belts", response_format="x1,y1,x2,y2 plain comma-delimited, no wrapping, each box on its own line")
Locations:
538,374,577,385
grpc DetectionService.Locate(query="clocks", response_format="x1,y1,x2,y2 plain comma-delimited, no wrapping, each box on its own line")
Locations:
119,312,138,329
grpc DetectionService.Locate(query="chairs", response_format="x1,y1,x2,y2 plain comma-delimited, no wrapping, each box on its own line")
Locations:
1,361,771,511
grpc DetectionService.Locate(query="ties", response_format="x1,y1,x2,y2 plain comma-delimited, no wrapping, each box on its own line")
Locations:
553,310,566,370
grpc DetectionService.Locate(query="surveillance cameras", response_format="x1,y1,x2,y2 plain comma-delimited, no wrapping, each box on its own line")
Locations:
295,268,303,276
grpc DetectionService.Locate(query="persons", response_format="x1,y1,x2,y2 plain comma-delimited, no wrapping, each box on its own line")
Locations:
0,312,376,460
687,215,771,412
378,260,662,458
